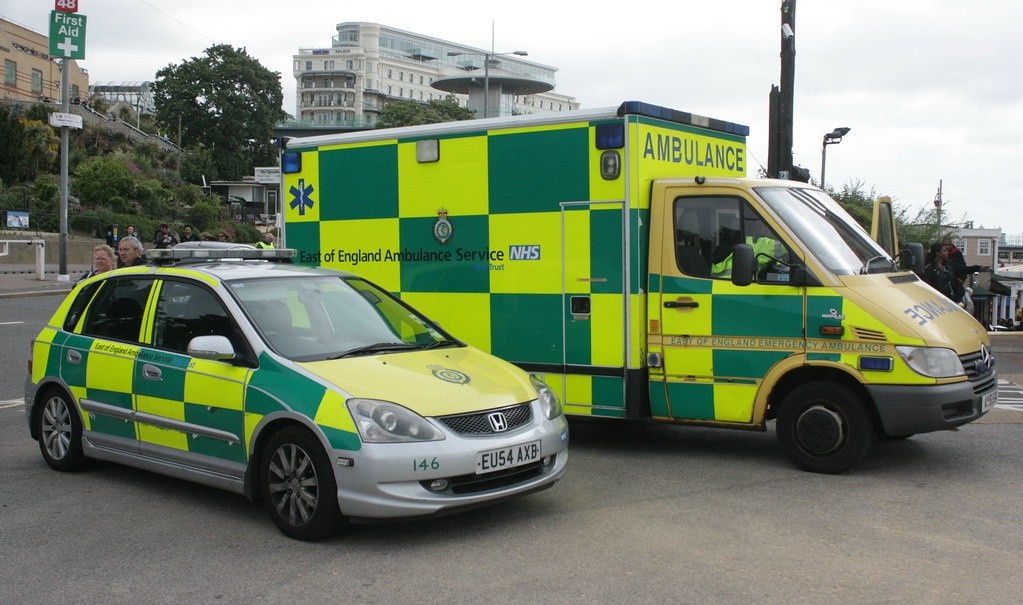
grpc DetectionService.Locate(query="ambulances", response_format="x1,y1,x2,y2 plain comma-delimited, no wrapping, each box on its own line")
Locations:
271,97,1001,477
24,241,570,543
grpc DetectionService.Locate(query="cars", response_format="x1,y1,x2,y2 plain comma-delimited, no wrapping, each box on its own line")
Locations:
170,240,268,263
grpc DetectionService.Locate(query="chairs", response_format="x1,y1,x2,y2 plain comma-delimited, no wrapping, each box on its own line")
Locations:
186,293,249,355
258,300,292,333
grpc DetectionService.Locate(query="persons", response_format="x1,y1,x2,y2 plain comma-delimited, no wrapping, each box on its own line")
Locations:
200,232,217,241
218,231,231,243
179,223,199,243
940,237,967,283
73,244,117,291
925,243,965,301
153,223,178,248
116,237,149,268
121,225,141,242
256,232,275,249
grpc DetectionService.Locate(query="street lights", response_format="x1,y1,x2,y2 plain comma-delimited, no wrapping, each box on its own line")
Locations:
54,80,60,104
933,179,944,244
136,92,143,129
447,49,530,118
819,127,851,190
